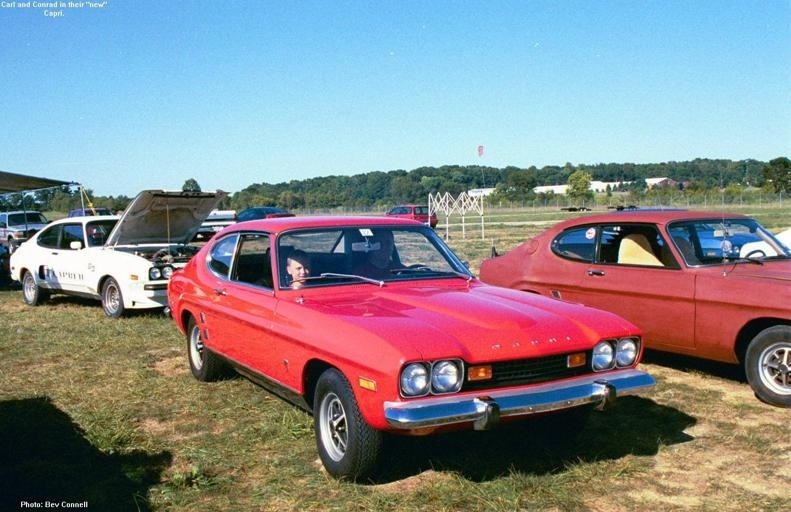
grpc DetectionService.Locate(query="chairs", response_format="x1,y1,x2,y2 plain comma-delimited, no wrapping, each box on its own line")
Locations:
616,233,702,267
265,246,293,287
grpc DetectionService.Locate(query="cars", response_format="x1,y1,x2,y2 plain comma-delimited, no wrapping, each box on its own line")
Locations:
0,189,790,482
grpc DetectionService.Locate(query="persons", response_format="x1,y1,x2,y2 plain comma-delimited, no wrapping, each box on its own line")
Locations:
281,249,316,289
344,230,410,281
